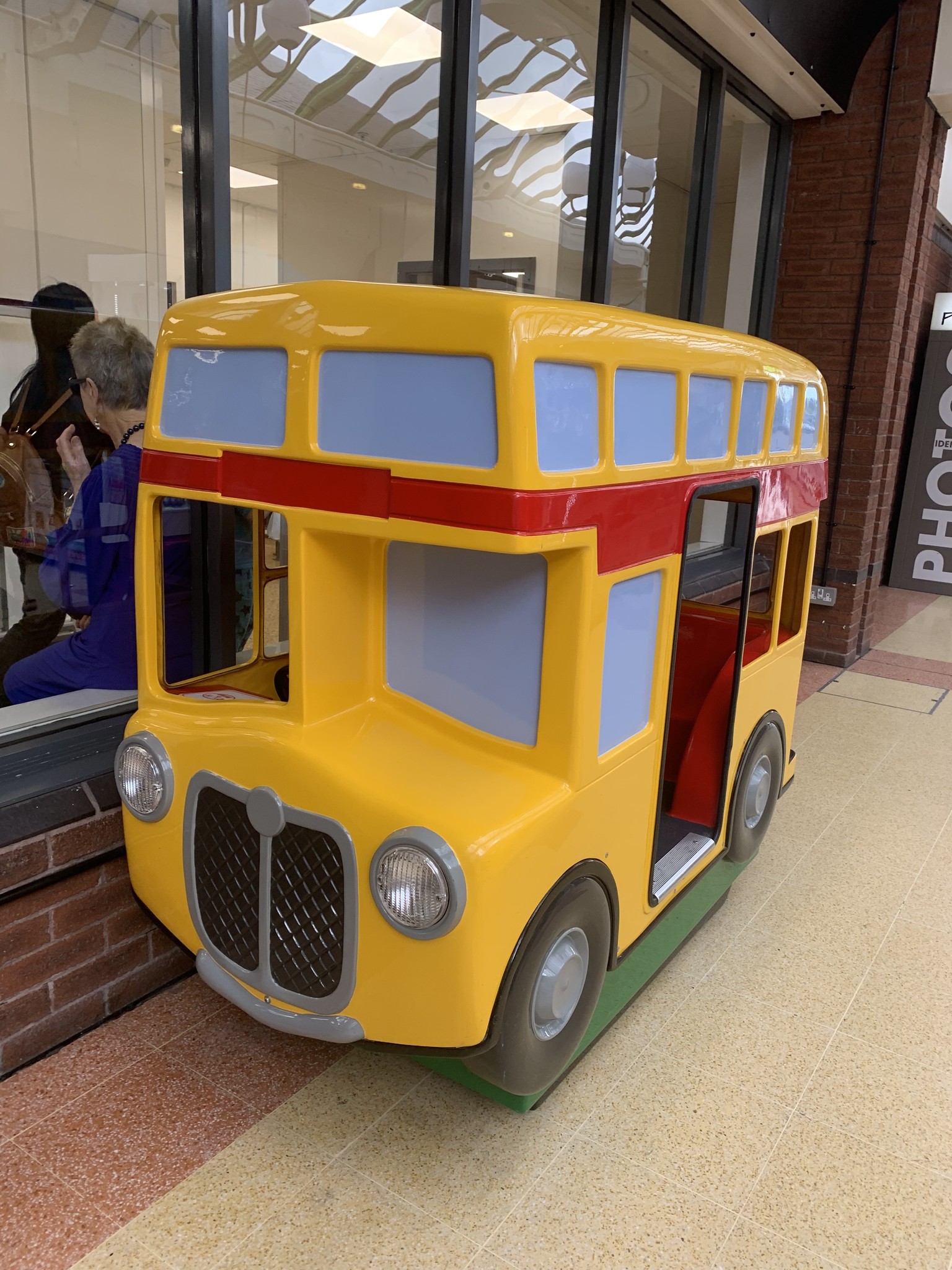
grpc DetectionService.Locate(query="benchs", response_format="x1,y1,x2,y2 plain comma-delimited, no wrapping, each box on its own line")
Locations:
667,607,792,825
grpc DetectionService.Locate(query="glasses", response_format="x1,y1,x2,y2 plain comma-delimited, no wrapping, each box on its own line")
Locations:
68,376,93,395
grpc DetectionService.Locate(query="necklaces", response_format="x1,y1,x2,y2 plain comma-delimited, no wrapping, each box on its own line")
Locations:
118,423,144,445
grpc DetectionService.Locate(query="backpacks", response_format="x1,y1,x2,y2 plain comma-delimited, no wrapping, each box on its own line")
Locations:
0,372,78,557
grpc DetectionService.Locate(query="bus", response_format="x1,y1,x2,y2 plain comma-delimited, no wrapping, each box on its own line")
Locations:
112,277,837,1099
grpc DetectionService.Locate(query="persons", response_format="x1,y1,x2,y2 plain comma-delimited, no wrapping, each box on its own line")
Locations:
0,282,115,706
0,315,256,708
